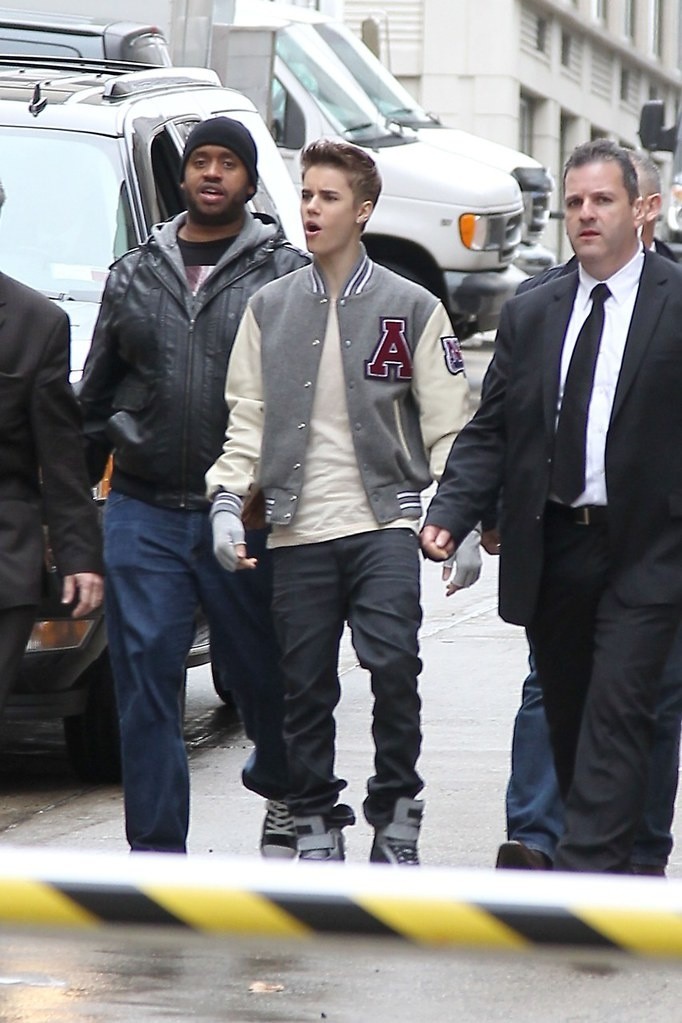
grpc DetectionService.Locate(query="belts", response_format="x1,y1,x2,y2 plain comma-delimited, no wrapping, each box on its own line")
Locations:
545,499,609,525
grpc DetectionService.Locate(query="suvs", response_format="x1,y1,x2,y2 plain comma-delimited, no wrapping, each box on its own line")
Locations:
0,14,306,776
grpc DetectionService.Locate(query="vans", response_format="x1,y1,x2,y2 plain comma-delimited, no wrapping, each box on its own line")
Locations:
234,8,556,341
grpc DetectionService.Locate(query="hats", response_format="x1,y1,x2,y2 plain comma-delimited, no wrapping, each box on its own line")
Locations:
180,117,258,204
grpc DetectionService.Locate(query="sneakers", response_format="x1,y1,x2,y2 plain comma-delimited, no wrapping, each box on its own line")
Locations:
261,799,299,859
371,796,426,866
291,813,345,864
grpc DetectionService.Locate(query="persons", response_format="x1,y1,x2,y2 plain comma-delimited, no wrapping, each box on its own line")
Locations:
202,137,484,865
0,181,104,730
414,138,681,885
73,117,309,860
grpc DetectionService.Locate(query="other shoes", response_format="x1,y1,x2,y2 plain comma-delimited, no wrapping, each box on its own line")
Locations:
497,842,548,870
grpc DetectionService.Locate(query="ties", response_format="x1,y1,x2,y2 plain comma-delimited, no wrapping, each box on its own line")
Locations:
549,284,614,505
